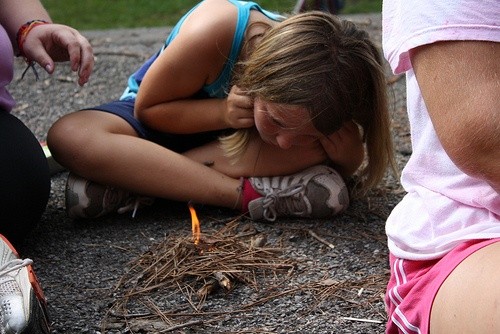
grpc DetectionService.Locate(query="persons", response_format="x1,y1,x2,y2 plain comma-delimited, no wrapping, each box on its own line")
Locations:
0,0,95,333
381,0,500,334
47,0,395,224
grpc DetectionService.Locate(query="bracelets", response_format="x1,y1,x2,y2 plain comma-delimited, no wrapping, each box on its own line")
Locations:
15,20,49,85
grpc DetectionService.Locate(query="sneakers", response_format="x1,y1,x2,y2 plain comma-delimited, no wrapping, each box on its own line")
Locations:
66,172,154,224
248,164,349,222
0,234,46,334
